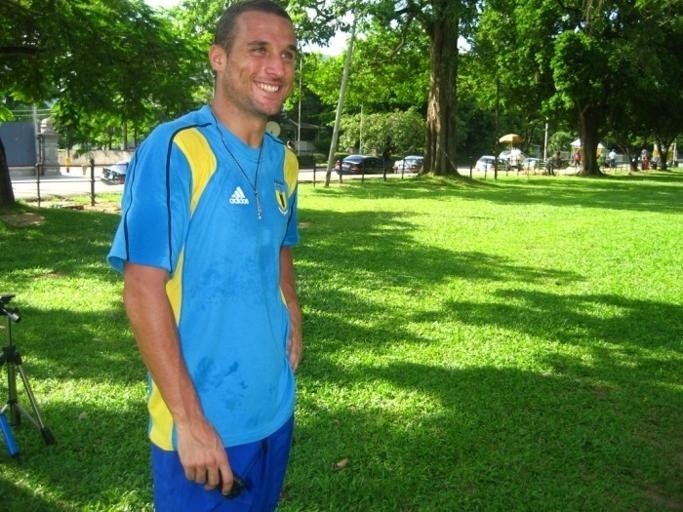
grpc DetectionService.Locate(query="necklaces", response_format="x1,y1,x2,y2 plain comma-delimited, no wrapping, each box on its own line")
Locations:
214,115,265,218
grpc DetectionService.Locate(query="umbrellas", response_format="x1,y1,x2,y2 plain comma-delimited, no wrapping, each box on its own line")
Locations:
499,132,523,150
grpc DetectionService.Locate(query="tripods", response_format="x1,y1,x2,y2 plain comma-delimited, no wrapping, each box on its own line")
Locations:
0,317,56,444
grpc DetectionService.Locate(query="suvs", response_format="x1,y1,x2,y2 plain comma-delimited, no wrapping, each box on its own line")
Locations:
497,148,530,171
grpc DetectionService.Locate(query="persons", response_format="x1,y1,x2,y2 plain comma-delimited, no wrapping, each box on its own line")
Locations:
105,1,302,512
545,148,617,176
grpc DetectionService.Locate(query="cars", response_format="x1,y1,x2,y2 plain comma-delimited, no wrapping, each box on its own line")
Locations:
335,154,393,175
101,160,131,186
394,155,425,174
521,157,542,172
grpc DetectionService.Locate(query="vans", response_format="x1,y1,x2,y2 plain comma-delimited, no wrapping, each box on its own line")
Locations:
474,154,511,173
